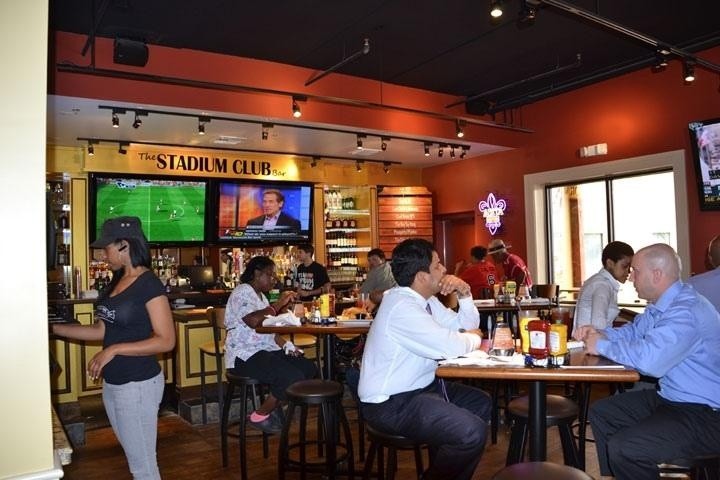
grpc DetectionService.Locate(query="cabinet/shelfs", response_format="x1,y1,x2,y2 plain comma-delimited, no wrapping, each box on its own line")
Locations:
322,185,372,282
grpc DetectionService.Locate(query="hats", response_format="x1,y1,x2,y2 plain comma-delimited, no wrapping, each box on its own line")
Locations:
487,239,511,255
89,217,141,249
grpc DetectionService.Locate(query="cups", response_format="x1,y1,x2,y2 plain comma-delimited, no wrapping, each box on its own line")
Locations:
550,307,570,343
517,310,540,355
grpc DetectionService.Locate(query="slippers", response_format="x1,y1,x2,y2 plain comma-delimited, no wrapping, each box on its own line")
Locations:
247,413,283,433
271,406,286,425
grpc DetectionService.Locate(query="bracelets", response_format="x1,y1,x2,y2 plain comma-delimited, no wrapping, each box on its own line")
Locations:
267,301,283,312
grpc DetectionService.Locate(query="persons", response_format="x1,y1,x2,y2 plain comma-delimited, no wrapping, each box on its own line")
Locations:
49,215,177,480
292,243,333,301
488,239,533,299
453,245,500,298
572,244,719,479
570,240,658,399
354,246,396,295
222,256,316,435
355,236,496,480
684,233,719,308
244,187,304,237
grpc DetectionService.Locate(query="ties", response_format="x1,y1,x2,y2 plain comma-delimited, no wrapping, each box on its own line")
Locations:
426,304,449,403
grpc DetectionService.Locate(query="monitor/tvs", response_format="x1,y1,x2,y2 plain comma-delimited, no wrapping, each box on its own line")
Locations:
86,170,210,250
686,118,720,211
210,175,315,248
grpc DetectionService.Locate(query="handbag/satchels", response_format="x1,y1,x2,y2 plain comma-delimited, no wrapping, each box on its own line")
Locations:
331,334,366,367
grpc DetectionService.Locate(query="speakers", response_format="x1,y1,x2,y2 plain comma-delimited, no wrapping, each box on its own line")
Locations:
113,37,150,67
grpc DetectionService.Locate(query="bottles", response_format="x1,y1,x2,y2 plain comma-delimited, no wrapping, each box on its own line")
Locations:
323,189,368,284
291,301,304,319
87,254,177,291
488,316,568,369
310,287,336,324
227,251,302,288
491,280,531,305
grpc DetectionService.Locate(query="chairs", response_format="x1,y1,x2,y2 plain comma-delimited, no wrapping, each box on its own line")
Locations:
197,294,322,423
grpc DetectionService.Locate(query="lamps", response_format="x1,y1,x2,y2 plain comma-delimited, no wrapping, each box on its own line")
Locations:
650,42,696,86
455,120,467,138
111,108,148,130
422,140,471,159
488,0,539,32
356,133,391,151
198,116,211,135
261,122,273,140
292,95,308,118
